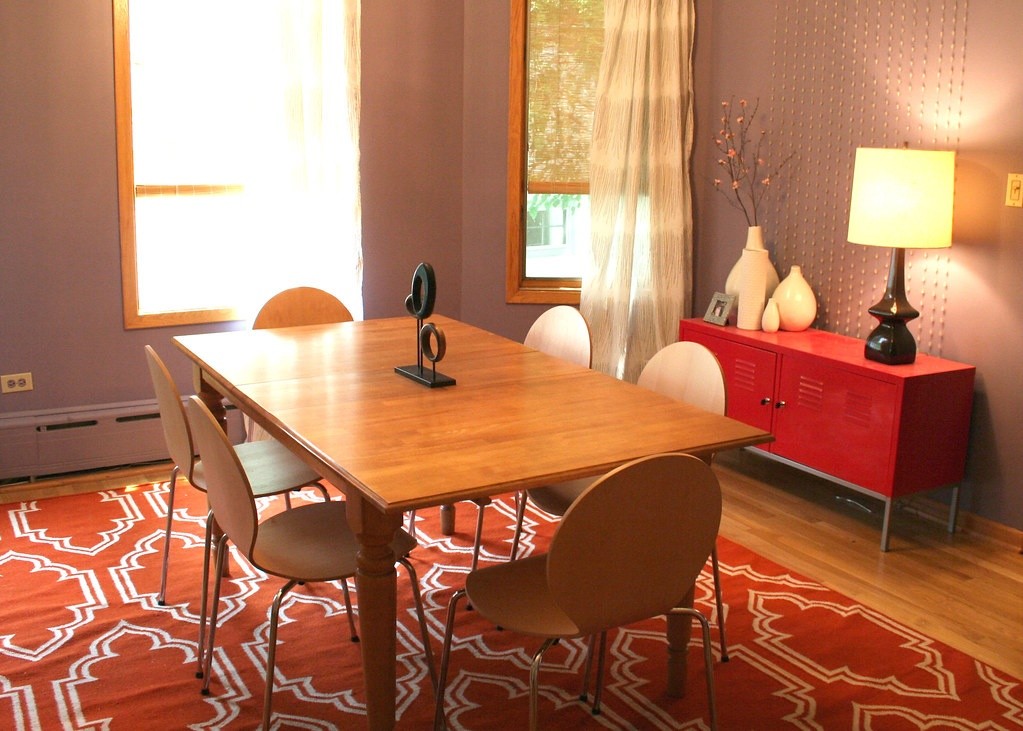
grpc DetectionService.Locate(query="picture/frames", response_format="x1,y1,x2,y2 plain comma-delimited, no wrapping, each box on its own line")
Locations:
703,293,737,327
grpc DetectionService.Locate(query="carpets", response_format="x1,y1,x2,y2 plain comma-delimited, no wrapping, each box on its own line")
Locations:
0,477,1023,731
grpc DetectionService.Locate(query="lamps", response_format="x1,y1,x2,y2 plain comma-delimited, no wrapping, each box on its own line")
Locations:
847,146,955,365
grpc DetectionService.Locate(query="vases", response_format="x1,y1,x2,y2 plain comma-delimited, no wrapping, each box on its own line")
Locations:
771,265,817,331
724,224,780,324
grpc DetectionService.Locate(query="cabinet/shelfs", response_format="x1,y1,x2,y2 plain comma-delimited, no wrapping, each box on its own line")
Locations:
678,318,977,552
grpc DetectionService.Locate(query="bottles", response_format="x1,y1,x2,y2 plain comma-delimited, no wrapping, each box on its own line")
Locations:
761,298,780,333
771,265,817,331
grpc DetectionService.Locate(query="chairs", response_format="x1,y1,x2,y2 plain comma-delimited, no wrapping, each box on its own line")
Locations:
252,286,354,330
493,341,729,664
433,453,723,731
143,343,333,679
403,305,594,612
186,394,439,731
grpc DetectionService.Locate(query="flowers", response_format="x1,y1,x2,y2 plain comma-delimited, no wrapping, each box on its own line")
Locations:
697,94,797,227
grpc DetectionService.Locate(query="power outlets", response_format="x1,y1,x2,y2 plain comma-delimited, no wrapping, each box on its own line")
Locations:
0,371,33,393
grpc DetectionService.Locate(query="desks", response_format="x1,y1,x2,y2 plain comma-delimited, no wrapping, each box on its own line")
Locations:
170,312,776,731
